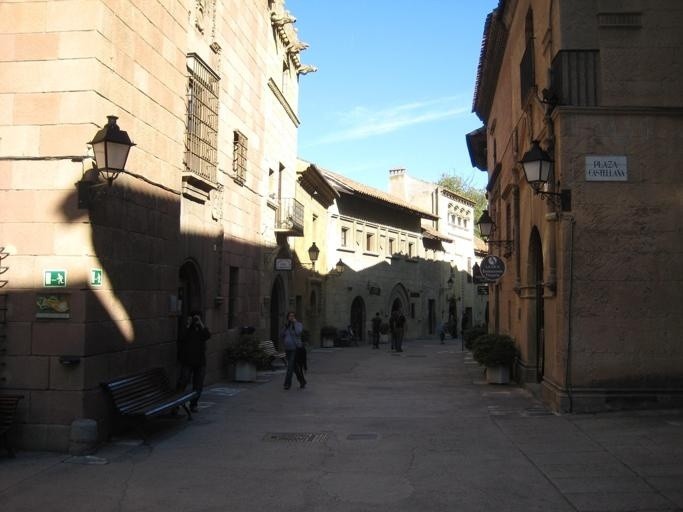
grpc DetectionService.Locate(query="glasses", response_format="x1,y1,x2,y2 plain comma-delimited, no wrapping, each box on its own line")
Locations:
521,139,571,213
76,118,132,207
476,210,512,254
307,240,319,271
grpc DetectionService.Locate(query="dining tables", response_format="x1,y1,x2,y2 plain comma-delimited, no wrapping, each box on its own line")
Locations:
224,327,256,382
473,335,514,385
320,325,337,348
379,322,389,344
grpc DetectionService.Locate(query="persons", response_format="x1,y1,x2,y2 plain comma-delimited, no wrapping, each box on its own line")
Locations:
388,309,400,350
370,312,382,350
393,311,408,353
278,311,308,392
169,308,211,417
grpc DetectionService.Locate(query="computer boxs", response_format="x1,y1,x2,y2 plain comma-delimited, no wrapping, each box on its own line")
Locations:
546,211,559,221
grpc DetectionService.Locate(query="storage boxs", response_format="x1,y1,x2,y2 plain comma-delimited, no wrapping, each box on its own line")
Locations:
106,365,197,439
0,390,31,460
257,340,287,371
